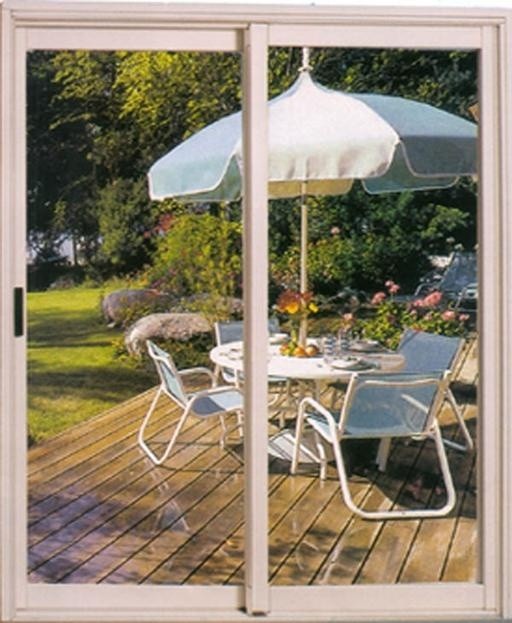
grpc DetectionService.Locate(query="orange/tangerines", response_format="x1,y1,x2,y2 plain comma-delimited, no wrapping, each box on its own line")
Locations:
281,335,317,357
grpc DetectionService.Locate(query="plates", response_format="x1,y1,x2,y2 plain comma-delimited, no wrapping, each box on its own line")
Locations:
345,343,384,353
330,357,372,370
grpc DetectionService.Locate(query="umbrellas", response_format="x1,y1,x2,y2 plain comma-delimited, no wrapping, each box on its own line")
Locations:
146,47,480,439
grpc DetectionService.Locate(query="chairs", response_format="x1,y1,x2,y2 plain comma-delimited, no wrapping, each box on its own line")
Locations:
289,369,456,519
138,339,247,466
213,326,476,454
